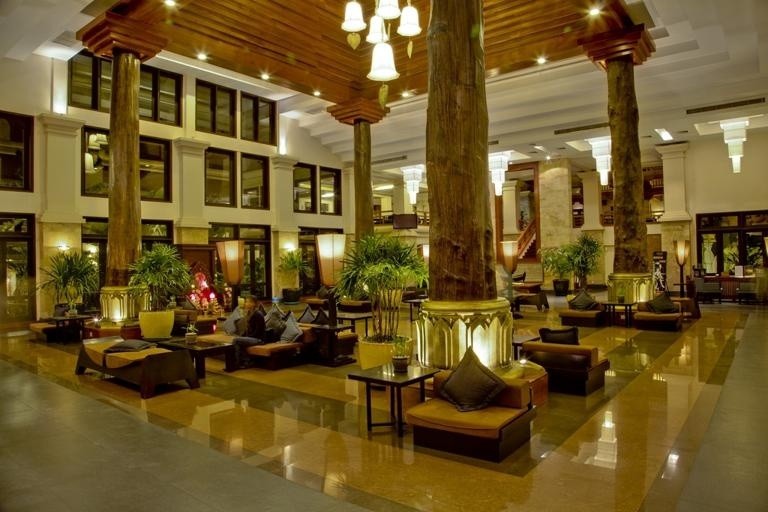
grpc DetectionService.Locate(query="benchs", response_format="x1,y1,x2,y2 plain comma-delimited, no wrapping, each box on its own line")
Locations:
196,317,305,370
28,315,200,399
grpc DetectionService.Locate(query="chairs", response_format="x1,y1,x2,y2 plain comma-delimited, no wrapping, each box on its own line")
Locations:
519,326,610,398
559,271,768,332
404,374,538,465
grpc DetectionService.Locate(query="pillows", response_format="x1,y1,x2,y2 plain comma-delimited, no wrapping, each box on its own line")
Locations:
512,270,526,282
223,300,330,345
438,347,508,412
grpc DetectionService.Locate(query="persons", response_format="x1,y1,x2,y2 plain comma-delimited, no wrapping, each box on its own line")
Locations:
234,295,266,369
654,262,664,292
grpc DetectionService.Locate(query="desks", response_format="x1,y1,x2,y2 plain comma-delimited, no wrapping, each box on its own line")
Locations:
512,280,548,312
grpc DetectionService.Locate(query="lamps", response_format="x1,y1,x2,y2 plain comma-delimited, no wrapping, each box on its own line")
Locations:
340,0,423,81
718,116,748,177
673,239,691,298
487,150,514,197
401,164,426,204
215,241,246,311
314,233,346,328
584,135,616,188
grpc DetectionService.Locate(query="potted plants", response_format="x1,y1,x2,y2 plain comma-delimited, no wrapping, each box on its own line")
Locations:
536,232,606,296
68,300,78,319
388,333,410,374
126,242,192,341
331,229,430,371
22,250,102,320
276,248,314,305
